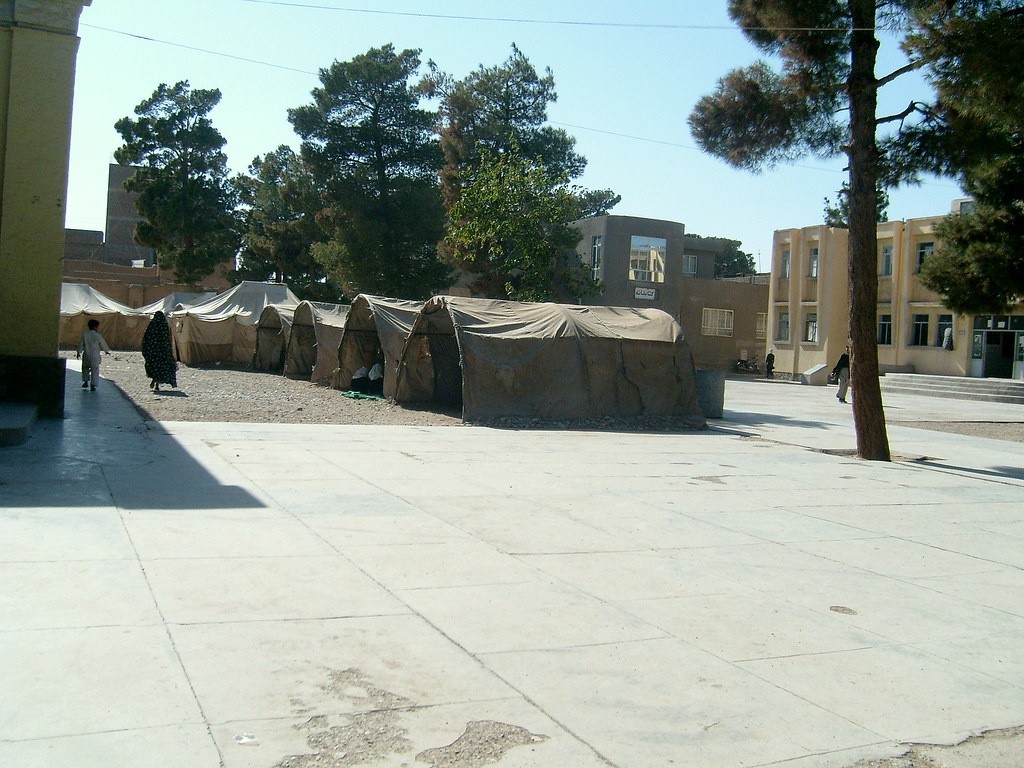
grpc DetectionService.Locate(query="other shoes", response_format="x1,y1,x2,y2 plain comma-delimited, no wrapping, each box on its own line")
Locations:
839,398,848,403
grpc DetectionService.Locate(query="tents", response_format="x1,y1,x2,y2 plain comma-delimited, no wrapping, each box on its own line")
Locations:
53,278,710,432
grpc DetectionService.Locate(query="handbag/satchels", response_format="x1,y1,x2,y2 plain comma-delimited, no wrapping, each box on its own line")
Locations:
831,372,838,384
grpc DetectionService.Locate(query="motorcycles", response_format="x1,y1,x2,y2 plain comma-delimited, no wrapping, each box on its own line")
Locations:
736,354,760,373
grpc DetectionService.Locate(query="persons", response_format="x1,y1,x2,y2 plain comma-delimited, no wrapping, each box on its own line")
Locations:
830,345,851,403
765,348,776,379
367,359,386,394
142,311,177,390
351,361,372,394
77,319,110,390
